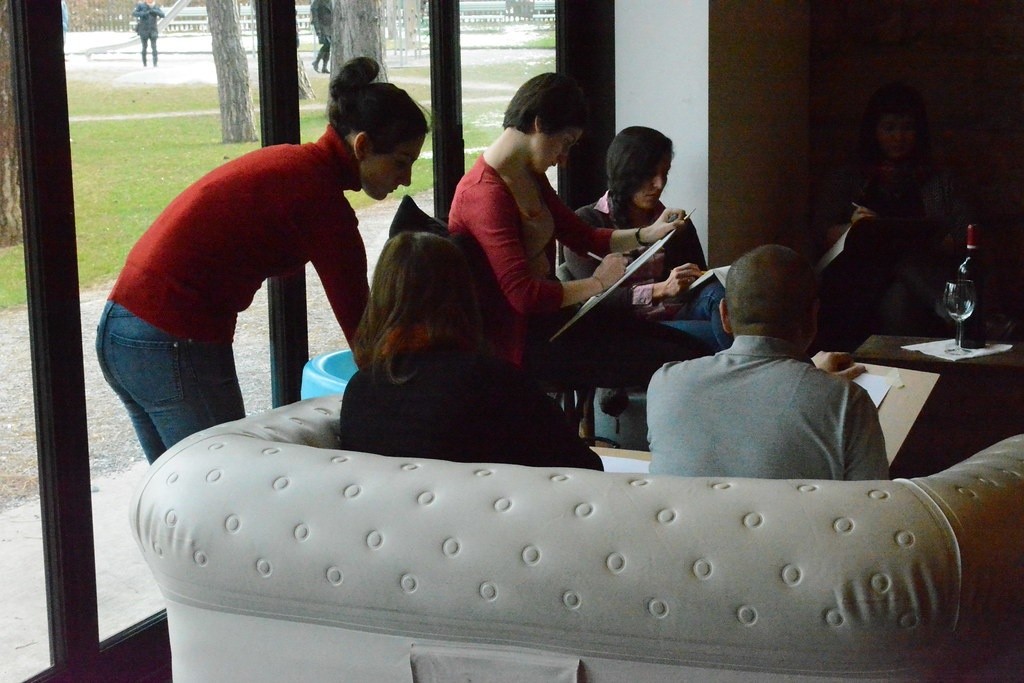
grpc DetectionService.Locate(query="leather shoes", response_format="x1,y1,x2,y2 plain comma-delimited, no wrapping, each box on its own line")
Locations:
311,61,331,73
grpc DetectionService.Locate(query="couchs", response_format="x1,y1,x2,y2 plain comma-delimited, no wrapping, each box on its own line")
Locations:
133,395,1023,682
554,261,723,454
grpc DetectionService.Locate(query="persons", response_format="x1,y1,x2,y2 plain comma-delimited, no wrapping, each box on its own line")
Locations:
310,0,334,74
94,56,434,468
562,126,736,353
646,244,891,482
448,72,717,391
131,0,166,68
809,81,969,355
338,232,605,472
60,0,70,62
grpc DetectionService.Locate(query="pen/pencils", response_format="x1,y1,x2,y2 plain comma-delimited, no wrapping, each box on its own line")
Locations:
588,251,603,262
851,202,860,207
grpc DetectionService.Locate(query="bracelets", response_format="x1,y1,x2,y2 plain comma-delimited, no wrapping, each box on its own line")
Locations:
590,275,604,297
635,227,653,247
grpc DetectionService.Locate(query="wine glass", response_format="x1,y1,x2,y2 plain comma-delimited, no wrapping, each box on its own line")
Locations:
944,279,975,354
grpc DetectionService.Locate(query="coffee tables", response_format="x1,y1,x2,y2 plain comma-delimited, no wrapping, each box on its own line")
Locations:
850,334,1024,458
